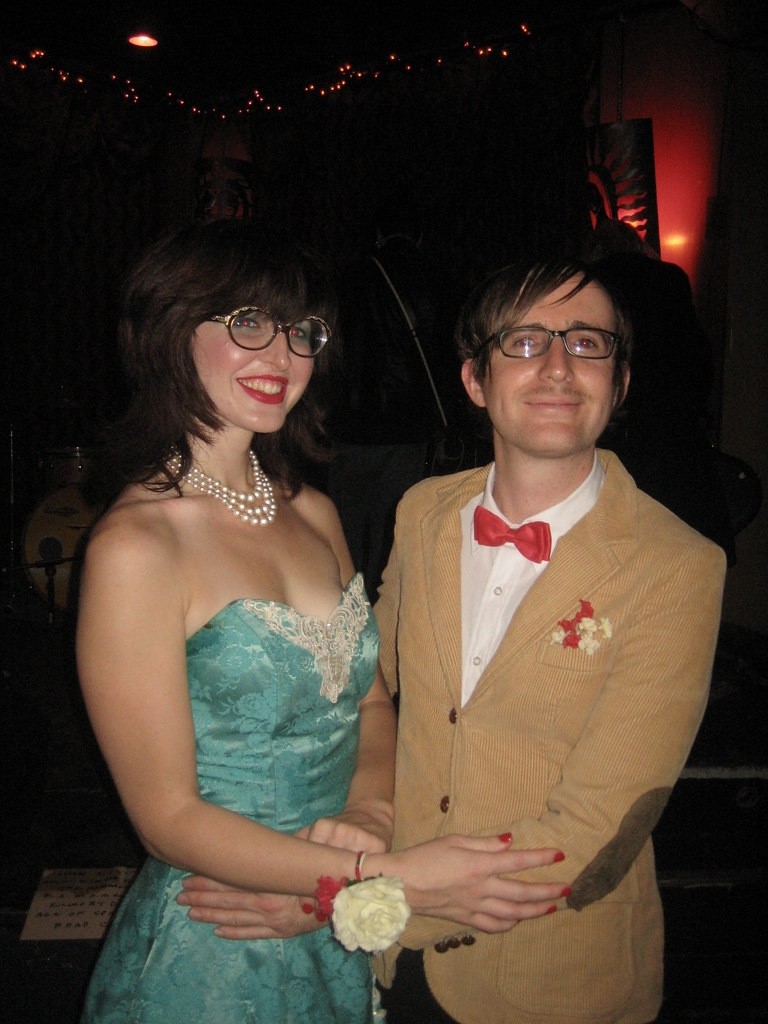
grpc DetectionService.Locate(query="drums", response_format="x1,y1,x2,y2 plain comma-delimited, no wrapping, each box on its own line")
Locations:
53,446,91,490
20,485,111,617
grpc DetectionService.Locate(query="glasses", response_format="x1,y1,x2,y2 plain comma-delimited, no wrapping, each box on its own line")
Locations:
473,326,621,359
208,307,336,358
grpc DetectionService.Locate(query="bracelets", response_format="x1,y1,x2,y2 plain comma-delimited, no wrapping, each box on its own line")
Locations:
303,850,410,952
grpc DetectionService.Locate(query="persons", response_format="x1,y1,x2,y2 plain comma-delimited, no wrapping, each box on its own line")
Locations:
175,261,727,1024
75,218,574,1024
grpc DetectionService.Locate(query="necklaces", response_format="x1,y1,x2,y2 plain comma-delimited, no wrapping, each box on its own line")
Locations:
164,447,277,526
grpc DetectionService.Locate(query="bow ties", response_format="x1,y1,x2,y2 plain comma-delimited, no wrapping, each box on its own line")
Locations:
474,505,552,564
